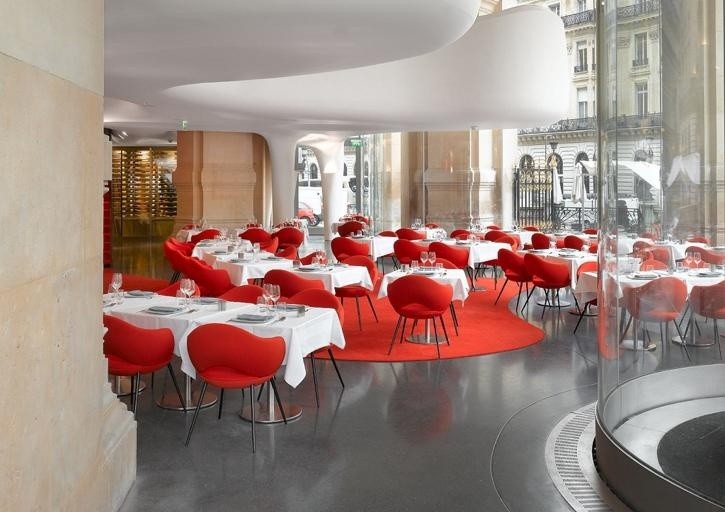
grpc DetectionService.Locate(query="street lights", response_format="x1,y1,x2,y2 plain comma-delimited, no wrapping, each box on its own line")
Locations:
544,134,559,205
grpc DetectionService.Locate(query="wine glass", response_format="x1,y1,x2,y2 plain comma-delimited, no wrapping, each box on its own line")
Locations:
102,273,286,316
398,250,446,278
308,248,336,273
337,209,491,247
190,216,303,265
510,218,723,282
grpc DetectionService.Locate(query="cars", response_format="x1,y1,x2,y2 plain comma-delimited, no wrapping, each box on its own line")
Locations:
297,174,371,228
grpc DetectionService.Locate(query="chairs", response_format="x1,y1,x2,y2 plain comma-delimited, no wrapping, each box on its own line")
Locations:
286,288,345,409
216,285,276,400
184,323,287,452
148,279,201,391
105,314,186,420
